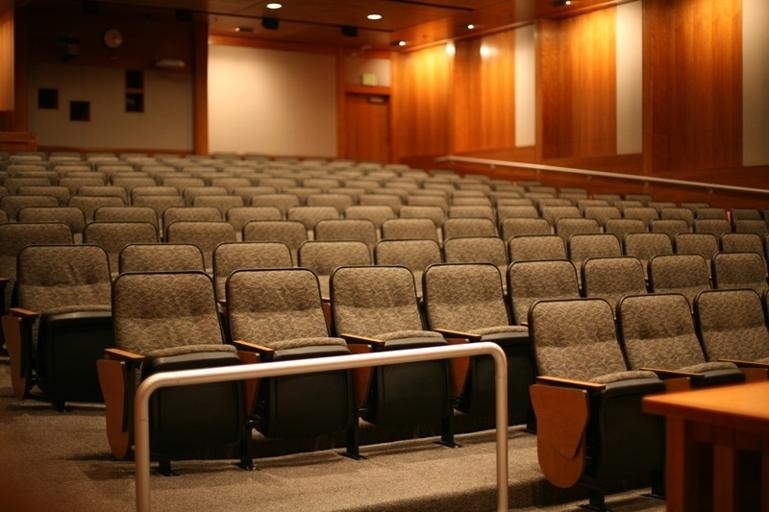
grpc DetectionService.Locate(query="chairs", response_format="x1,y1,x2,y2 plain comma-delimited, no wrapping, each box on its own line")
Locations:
0,150,769,512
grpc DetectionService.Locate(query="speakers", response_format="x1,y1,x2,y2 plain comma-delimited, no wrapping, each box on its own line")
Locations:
342,26,358,36
262,18,278,29
